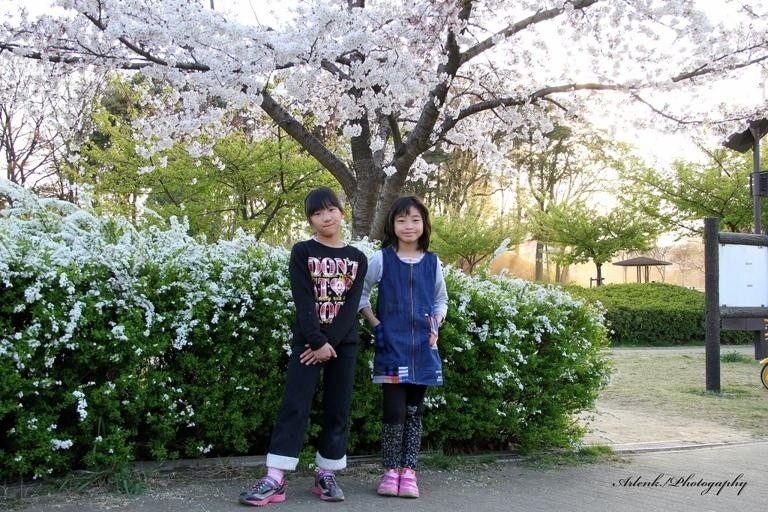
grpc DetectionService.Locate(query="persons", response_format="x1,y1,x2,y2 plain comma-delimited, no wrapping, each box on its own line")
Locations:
354,195,449,498
238,186,368,507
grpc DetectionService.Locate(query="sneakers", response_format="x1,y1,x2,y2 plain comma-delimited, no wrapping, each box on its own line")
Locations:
376,468,399,497
312,466,345,502
238,474,287,506
399,467,420,498
